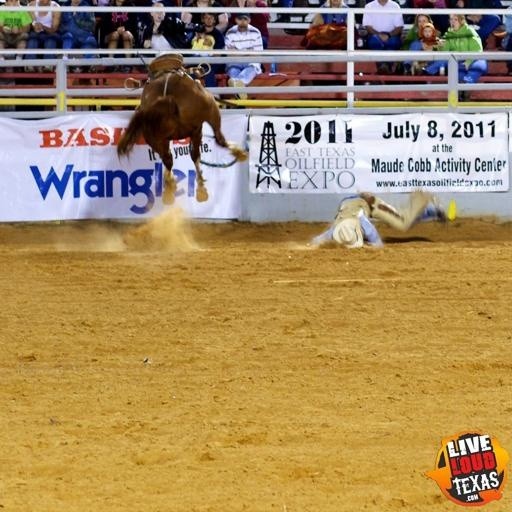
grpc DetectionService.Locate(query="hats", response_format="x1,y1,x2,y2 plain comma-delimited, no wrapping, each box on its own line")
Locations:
332,218,365,249
421,23,435,30
236,13,250,20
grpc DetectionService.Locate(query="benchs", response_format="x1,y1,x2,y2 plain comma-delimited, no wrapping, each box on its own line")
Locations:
0,0,511,106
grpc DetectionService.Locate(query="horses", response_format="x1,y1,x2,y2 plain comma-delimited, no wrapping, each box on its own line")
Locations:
116,67,249,204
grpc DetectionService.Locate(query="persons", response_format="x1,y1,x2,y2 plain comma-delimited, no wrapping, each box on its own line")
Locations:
311,191,448,249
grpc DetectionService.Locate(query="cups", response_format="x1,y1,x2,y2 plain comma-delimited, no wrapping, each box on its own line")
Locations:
439,66,446,76
270,63,277,73
